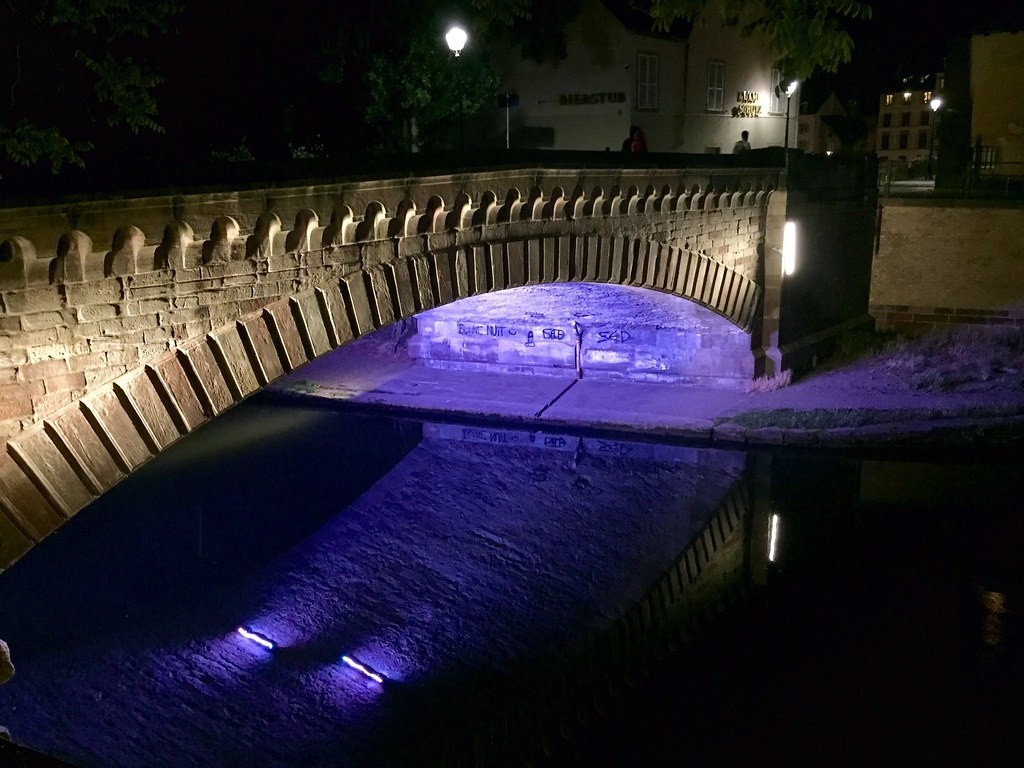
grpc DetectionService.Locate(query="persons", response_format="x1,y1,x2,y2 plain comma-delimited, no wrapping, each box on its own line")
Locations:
619,125,650,171
731,131,752,156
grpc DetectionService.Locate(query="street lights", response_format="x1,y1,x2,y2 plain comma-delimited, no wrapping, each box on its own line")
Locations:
928,96,942,181
782,79,800,166
444,23,473,172
